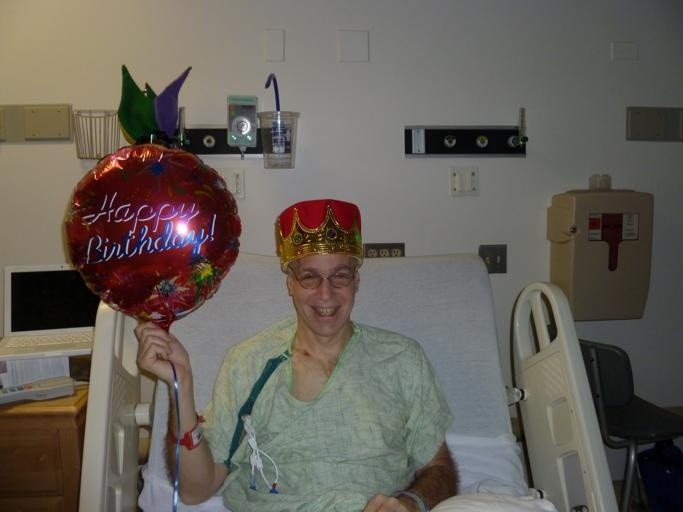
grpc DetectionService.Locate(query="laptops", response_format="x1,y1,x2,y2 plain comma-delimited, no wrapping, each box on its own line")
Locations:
0,263,102,357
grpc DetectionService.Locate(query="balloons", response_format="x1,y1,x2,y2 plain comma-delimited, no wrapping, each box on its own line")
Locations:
60,142,242,325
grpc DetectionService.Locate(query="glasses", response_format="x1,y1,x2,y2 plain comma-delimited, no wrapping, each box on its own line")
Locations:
288,263,358,290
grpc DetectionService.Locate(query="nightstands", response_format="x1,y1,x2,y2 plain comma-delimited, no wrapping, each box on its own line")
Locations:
0,386,90,512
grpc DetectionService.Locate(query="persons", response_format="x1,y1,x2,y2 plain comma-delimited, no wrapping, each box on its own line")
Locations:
131,197,460,512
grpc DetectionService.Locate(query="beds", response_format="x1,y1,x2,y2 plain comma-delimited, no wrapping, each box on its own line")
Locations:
78,252,616,512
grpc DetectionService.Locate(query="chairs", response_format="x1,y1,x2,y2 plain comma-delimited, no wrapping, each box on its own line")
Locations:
578,338,683,512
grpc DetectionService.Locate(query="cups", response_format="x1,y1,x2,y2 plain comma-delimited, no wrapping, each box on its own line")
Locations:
259,110,299,171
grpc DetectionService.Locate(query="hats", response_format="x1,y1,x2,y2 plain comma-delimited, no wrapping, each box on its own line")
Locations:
274,199,363,274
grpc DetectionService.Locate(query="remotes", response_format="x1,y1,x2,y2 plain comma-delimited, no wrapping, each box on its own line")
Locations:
0,374,75,406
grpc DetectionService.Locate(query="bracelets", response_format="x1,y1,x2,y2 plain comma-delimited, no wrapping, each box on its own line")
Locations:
170,408,205,451
389,487,426,511
